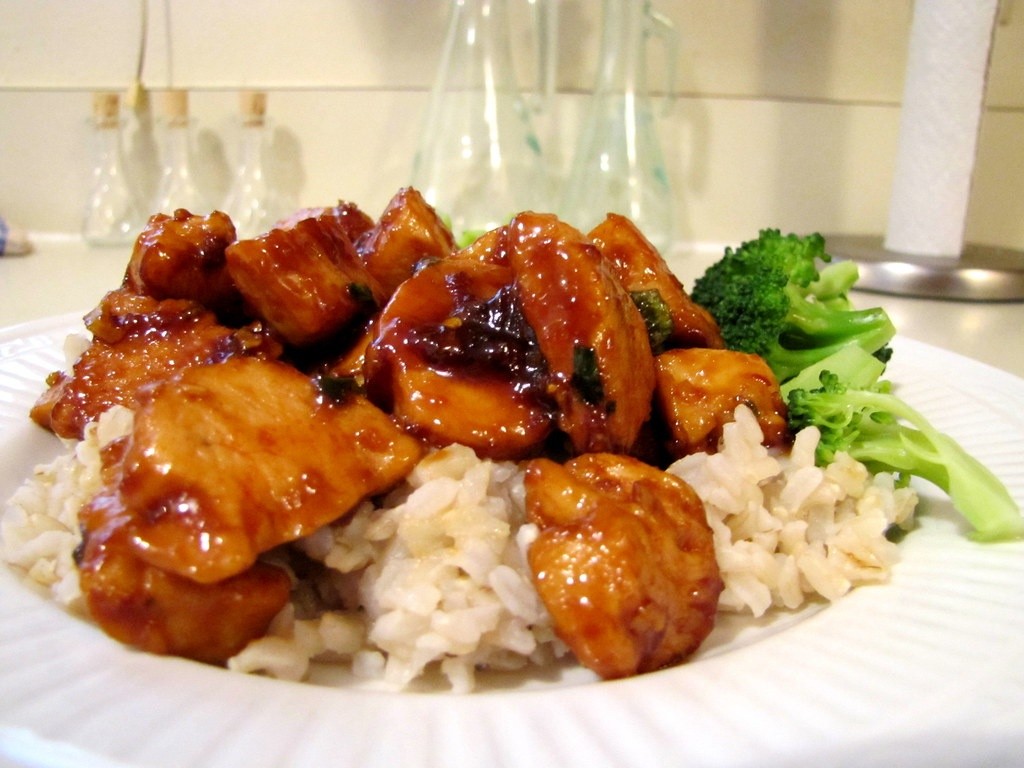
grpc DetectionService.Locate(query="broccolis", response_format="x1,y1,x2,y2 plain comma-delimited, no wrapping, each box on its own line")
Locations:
685,226,1024,543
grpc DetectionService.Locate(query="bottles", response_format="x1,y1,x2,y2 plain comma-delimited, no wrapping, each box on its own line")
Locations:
80,95,143,247
216,92,288,240
147,90,212,217
118,84,158,225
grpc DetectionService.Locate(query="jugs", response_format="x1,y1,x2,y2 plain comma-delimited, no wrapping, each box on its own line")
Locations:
415,1,685,259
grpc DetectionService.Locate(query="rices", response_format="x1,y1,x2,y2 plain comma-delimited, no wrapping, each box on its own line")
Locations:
0,334,920,693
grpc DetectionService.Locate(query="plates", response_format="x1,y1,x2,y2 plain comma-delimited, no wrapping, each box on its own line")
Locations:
0,305,1024,768
